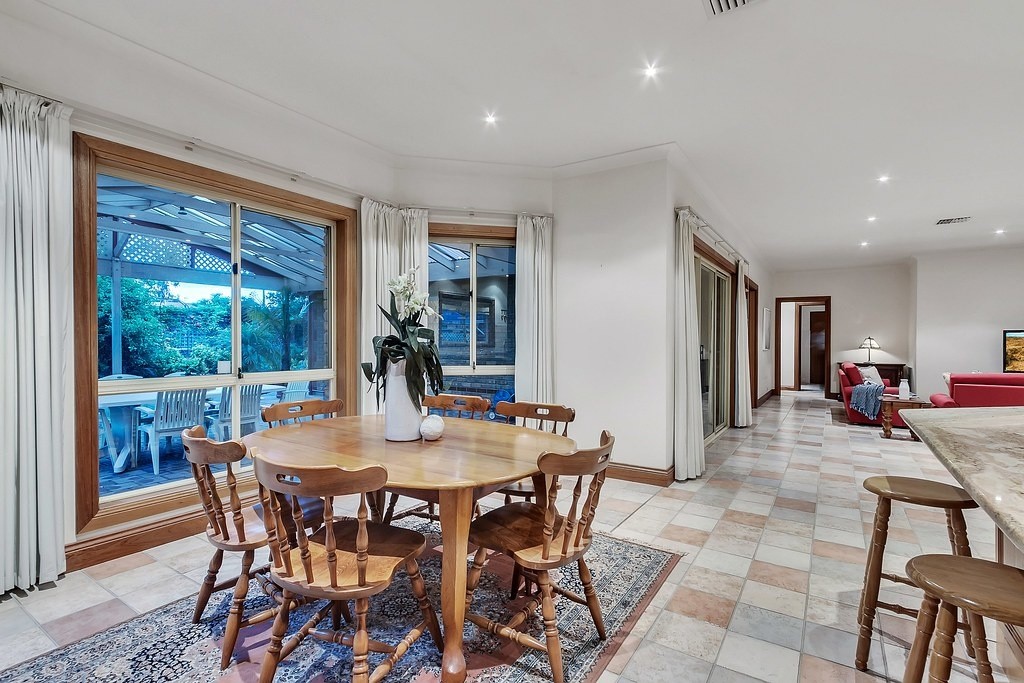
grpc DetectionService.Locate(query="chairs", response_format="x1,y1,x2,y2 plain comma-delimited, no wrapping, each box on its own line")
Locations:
98,380,614,683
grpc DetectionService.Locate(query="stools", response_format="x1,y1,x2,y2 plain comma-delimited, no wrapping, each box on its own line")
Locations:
903,554,1024,683
855,476,980,673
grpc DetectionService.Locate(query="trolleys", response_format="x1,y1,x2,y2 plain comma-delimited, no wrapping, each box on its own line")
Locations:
489,388,515,420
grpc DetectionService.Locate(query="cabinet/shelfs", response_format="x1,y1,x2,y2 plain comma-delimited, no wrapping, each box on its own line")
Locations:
837,362,906,402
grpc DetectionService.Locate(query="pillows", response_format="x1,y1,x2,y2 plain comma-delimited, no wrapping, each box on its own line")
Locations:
943,373,951,387
857,365,884,386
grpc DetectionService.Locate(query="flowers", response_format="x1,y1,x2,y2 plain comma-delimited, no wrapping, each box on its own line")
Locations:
353,264,452,416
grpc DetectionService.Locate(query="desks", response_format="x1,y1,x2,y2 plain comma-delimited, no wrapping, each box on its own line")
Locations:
241,416,577,682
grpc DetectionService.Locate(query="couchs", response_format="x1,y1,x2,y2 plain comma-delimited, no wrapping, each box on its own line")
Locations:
929,373,1024,408
839,362,900,425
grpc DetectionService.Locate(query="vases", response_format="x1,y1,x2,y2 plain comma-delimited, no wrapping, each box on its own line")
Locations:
899,378,910,400
386,358,429,441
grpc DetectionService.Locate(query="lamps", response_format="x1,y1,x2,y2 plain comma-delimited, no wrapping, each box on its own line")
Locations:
858,336,880,363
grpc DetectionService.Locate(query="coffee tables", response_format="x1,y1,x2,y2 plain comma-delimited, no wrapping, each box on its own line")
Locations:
876,394,926,441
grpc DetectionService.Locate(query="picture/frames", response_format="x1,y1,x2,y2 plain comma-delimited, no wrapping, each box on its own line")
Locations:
1002,329,1024,373
763,308,772,350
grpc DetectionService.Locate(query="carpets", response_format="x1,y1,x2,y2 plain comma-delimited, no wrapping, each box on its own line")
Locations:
0,522,679,683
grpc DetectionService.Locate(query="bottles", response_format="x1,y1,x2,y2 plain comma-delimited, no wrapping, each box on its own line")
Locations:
899,379,909,400
